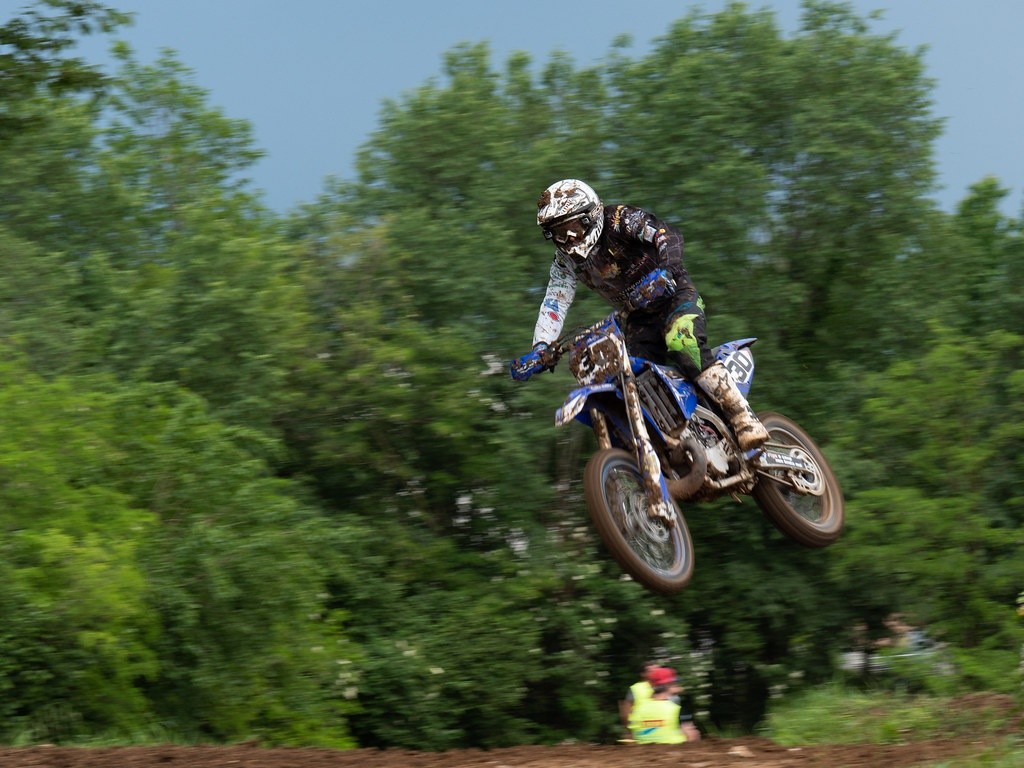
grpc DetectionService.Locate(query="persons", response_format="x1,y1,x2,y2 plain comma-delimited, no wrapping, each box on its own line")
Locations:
626,668,701,745
837,611,947,692
531,179,772,453
616,661,683,724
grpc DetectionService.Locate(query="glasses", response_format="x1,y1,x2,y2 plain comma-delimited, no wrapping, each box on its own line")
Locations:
544,212,594,245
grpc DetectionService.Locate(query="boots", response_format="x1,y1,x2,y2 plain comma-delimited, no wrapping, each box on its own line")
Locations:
695,360,771,451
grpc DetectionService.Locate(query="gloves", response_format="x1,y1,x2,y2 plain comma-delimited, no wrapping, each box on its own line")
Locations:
636,269,675,303
510,351,545,382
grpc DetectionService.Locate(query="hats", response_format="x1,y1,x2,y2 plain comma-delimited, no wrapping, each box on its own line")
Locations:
647,668,677,685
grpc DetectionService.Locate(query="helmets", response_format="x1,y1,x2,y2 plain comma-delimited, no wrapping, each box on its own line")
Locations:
537,178,605,262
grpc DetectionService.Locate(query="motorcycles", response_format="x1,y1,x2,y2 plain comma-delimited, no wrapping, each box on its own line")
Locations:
510,267,846,595
